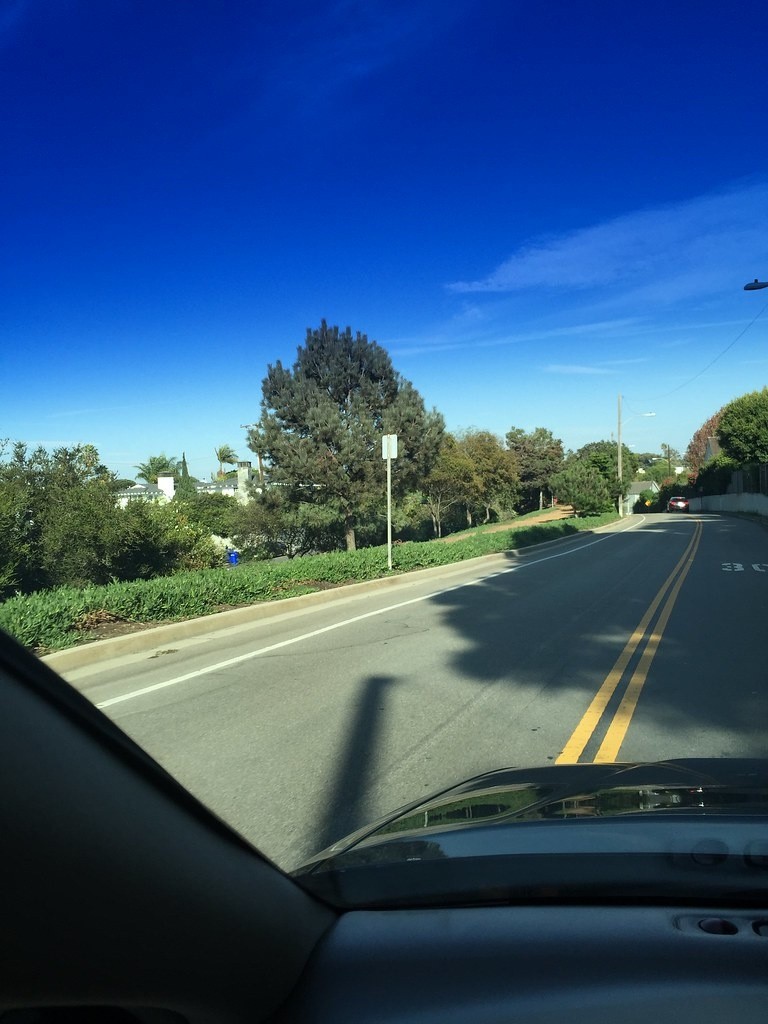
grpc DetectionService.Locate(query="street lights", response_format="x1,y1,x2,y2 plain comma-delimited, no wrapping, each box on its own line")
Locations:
652,456,671,477
617,411,656,519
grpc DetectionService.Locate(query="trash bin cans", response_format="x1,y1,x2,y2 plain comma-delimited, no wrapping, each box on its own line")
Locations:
554,497,558,503
228,552,239,565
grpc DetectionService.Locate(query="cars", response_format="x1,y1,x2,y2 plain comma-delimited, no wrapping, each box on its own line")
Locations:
668,497,690,514
